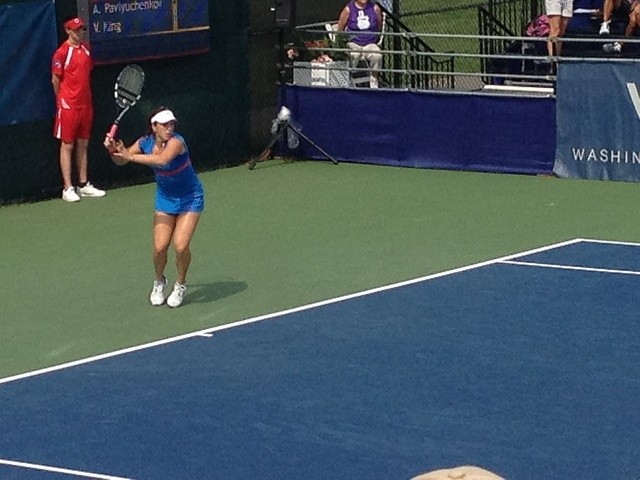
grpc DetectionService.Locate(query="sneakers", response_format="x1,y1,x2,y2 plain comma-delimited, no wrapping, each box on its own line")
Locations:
77,182,106,196
603,43,622,53
150,276,167,306
599,21,610,35
167,282,186,308
62,186,80,202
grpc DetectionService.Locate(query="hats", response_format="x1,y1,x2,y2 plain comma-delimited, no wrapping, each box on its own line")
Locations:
64,15,86,31
151,109,177,124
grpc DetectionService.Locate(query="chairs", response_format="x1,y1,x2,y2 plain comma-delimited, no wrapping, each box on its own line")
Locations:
566,0,640,36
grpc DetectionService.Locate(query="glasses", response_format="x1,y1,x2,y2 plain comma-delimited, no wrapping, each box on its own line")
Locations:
74,27,88,32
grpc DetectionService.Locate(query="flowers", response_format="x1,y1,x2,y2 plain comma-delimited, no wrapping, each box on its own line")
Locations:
286,32,353,62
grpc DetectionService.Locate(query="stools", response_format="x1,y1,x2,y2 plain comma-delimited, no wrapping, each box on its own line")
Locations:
325,23,372,88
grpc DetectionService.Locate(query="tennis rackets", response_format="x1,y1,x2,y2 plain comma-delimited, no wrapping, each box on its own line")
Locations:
109,64,147,137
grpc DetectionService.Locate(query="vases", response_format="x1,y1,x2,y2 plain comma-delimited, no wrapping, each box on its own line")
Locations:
293,59,349,87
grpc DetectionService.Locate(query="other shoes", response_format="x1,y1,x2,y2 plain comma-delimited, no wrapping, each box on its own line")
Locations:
370,75,378,88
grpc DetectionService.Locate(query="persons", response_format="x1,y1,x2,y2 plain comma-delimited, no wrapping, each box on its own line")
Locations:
545,0,640,72
51,18,106,201
103,107,203,307
338,0,383,88
